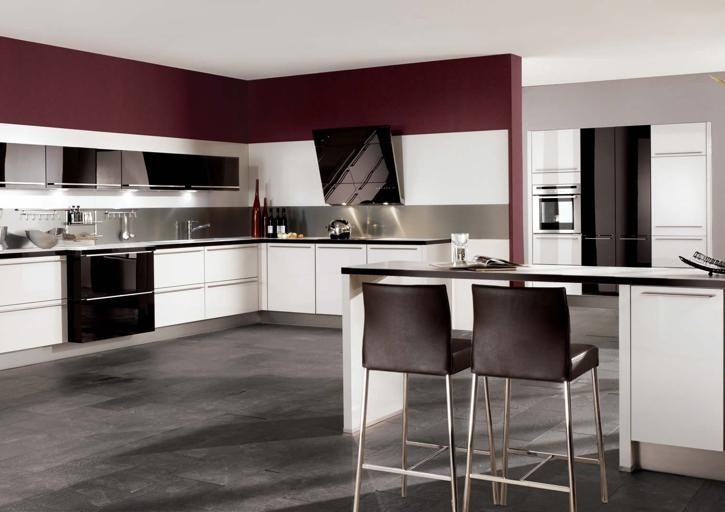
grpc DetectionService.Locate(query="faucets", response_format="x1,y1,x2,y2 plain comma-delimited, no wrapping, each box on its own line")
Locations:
187,220,211,240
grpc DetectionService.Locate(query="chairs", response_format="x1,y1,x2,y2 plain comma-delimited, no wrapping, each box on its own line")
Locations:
353,282,500,512
463,285,607,512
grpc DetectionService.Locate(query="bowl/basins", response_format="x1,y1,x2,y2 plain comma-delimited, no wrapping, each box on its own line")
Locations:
25,227,65,250
0,225,7,250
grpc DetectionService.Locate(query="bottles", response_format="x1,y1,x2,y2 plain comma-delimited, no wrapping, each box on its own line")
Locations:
251,178,261,238
266,208,288,237
69,206,82,223
261,198,268,238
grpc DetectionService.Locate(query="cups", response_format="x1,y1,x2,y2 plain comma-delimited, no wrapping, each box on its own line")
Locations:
450,233,469,265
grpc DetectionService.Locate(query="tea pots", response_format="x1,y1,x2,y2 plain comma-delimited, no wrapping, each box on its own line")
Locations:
325,218,351,240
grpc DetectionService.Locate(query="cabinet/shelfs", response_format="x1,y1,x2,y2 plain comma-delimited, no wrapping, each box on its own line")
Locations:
316,244,367,316
0,255,69,354
367,243,453,331
263,243,315,314
154,243,262,328
528,122,712,296
323,131,389,206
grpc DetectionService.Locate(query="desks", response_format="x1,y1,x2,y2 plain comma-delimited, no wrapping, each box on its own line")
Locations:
342,264,725,482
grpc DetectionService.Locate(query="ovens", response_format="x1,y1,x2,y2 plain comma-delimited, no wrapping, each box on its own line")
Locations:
68,247,155,343
531,193,583,234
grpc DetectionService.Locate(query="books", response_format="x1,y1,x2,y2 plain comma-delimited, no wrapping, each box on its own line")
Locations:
430,255,530,270
